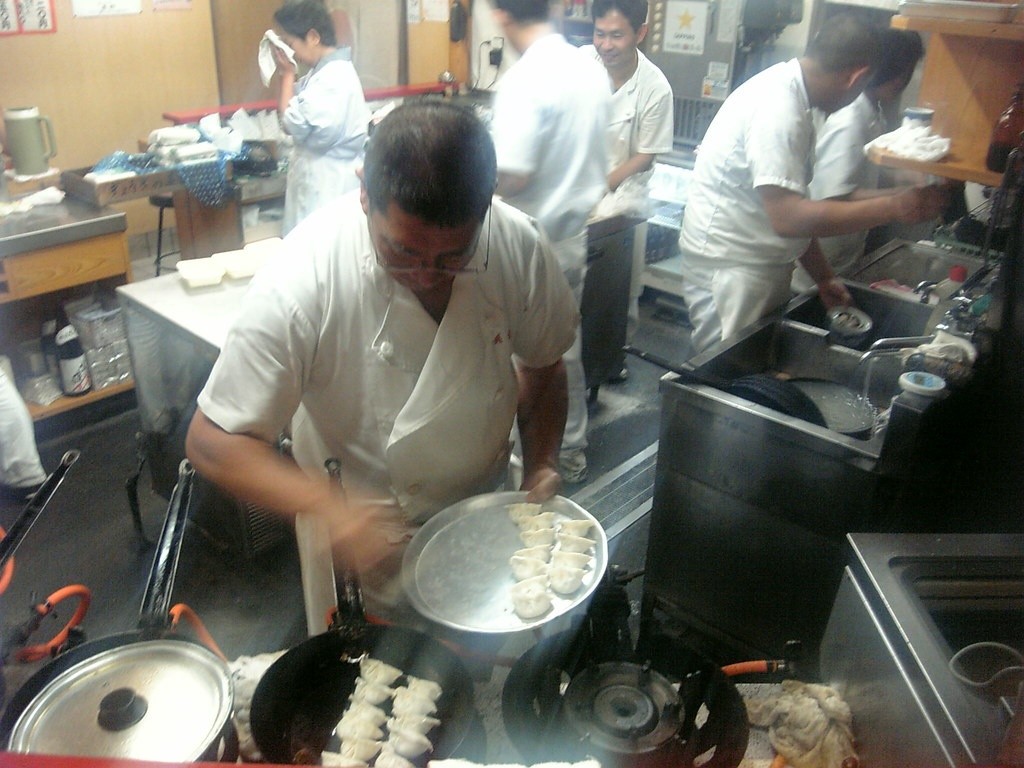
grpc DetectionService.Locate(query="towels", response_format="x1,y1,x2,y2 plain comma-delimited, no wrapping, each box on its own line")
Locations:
257,29,298,89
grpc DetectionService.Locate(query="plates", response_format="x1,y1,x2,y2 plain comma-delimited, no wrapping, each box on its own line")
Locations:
397,488,609,634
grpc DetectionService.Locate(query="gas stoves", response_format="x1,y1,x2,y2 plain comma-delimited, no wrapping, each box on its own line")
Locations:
0,563,750,768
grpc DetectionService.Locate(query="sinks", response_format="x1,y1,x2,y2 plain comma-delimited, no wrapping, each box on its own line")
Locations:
850,243,988,300
821,528,1024,768
782,284,934,355
675,322,906,441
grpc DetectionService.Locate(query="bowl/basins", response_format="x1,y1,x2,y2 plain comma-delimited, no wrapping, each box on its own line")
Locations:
786,376,879,437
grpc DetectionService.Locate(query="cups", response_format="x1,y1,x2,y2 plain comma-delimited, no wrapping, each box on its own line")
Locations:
902,106,934,132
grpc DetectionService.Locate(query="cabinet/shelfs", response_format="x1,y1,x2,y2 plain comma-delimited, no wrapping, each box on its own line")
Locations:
0,229,137,419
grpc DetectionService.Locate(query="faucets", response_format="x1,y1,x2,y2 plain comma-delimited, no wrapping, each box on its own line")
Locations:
912,278,937,304
857,335,940,366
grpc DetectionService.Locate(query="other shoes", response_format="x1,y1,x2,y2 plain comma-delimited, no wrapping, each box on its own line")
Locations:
557,448,587,483
604,358,629,384
0,482,46,505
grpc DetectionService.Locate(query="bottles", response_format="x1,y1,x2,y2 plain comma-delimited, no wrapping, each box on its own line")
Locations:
919,264,968,335
51,306,91,397
985,83,1024,172
37,294,60,380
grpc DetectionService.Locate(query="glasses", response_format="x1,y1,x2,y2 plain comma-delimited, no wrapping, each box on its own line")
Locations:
367,204,491,283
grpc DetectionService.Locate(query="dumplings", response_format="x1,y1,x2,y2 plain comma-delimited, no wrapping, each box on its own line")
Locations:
321,657,443,768
504,503,596,618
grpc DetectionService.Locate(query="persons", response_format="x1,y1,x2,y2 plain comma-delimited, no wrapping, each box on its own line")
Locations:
678,9,954,354
475,1,609,492
184,96,583,637
0,363,57,500
579,0,673,383
258,0,373,237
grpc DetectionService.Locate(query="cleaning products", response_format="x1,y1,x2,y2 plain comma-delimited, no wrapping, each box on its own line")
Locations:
922,262,970,336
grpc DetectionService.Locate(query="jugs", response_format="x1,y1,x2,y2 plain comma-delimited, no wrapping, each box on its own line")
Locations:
3,106,57,174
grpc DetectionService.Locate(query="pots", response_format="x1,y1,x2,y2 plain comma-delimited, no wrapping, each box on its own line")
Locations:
623,343,826,428
248,457,475,767
5,458,237,762
